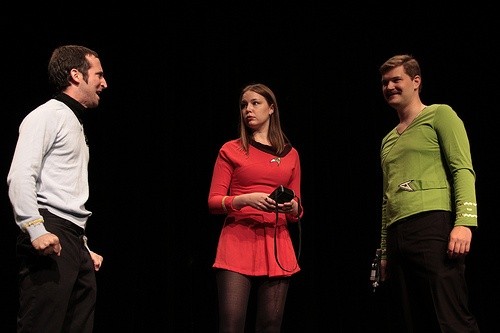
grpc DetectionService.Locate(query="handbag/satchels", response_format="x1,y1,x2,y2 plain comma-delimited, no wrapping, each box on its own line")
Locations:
270,185,295,206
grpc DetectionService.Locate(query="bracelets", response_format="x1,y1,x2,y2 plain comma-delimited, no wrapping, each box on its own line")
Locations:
221,196,230,215
21,218,44,229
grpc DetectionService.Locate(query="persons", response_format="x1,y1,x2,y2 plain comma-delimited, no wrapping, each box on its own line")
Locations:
377,54,478,333
6,45,107,333
207,84,304,333
